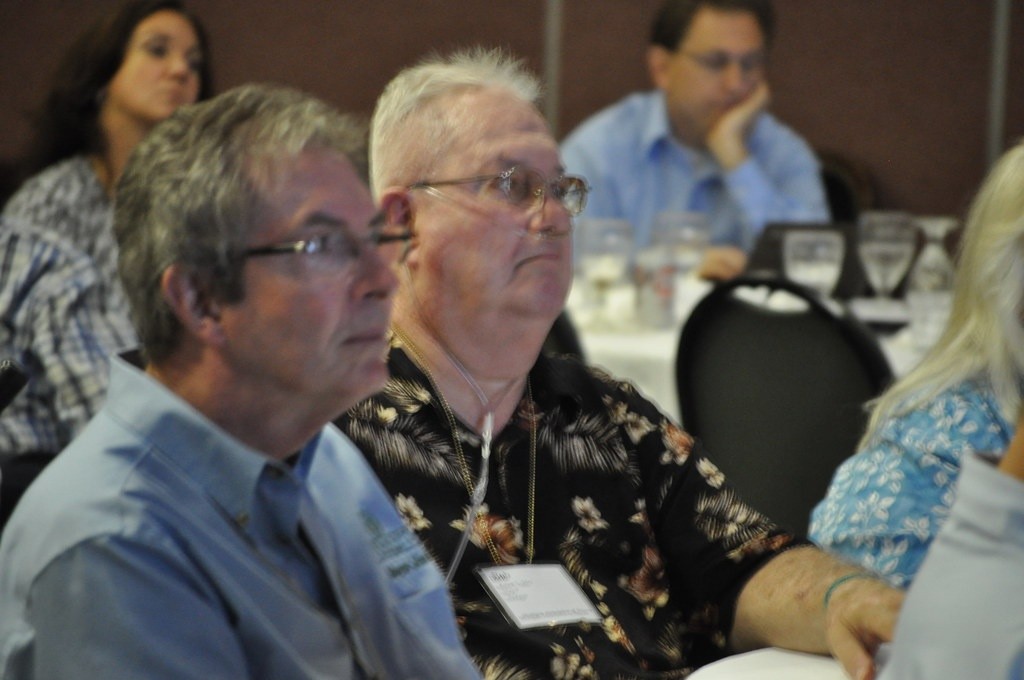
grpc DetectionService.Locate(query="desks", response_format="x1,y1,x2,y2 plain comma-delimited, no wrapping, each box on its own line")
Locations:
565,281,957,429
682,645,849,680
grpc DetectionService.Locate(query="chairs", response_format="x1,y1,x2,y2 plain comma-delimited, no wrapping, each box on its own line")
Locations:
675,279,900,549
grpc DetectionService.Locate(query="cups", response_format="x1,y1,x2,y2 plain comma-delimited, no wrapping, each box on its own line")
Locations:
783,230,844,294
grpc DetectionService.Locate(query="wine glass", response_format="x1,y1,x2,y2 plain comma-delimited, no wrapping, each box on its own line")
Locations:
908,214,959,307
856,209,917,310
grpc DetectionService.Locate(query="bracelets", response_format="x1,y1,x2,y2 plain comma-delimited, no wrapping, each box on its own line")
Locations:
825,573,861,606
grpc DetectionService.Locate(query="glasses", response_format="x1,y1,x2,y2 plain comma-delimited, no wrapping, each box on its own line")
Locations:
246,222,414,277
405,167,591,214
678,47,769,70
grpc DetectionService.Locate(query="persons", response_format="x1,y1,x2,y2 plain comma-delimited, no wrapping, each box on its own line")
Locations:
0,0,215,537
0,82,481,680
556,0,833,242
331,45,905,680
806,149,1024,680
